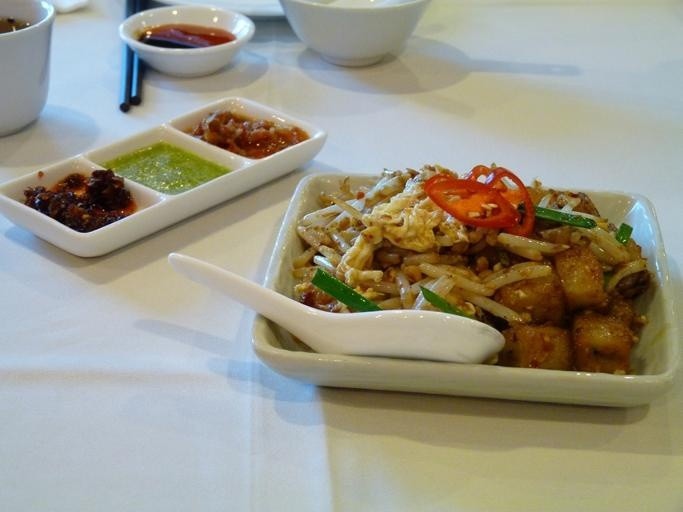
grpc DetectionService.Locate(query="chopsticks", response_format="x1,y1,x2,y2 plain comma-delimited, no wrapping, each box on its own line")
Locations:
118,0,145,113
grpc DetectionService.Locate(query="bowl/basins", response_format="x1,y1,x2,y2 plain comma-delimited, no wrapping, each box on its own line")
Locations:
279,0,431,67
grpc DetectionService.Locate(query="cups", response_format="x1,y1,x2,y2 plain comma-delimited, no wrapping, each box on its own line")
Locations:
0,0,56,138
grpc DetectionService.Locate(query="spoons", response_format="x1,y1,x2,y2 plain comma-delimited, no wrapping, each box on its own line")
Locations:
167,251,506,363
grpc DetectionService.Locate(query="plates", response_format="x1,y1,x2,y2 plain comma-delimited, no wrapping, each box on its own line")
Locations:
0,94,327,259
248,170,681,409
118,6,255,75
